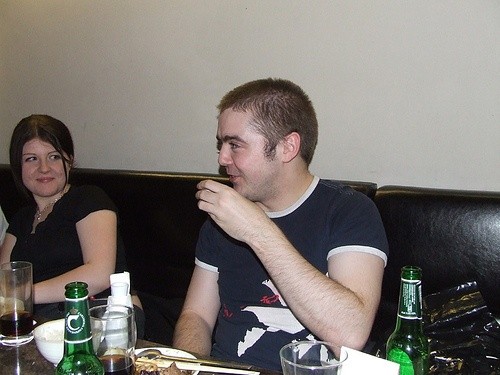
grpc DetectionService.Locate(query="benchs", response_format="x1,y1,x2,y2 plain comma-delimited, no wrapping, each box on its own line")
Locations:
0,163,378,352
373,185,500,341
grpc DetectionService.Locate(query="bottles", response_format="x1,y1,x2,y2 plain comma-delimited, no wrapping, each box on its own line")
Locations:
102,282,134,339
55,281,105,375
386,266,430,375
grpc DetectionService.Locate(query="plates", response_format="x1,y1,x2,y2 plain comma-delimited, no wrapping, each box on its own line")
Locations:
135,347,200,375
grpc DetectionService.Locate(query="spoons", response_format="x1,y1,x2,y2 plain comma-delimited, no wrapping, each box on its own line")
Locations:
136,349,253,370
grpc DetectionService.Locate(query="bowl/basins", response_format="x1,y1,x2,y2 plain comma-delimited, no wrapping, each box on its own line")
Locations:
33,317,103,367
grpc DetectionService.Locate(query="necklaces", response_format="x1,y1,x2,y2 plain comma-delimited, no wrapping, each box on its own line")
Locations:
36,196,62,221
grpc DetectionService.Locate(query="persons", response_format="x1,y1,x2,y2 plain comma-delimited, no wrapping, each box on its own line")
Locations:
173,77,387,375
0,115,145,339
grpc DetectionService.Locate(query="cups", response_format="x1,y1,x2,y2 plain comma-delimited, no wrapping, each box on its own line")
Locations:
0,261,34,346
279,341,348,375
89,305,136,375
